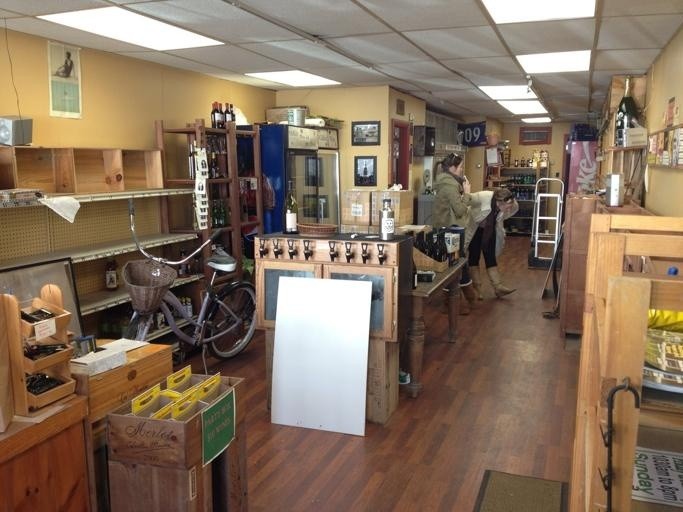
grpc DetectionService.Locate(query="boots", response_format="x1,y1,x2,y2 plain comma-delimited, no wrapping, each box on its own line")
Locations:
441,266,517,314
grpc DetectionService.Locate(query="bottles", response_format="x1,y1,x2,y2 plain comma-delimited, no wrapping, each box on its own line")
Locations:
613,73,639,146
513,155,531,168
378,191,394,241
98,295,196,339
210,151,219,179
210,100,235,132
506,173,535,184
105,258,121,291
404,229,448,264
176,247,191,280
503,186,534,200
208,199,225,228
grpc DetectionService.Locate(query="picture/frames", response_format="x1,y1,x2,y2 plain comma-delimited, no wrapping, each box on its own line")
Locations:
649,121,682,168
352,156,377,187
350,120,381,147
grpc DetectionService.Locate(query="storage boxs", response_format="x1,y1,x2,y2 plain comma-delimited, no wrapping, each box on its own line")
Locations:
340,189,370,226
370,190,416,224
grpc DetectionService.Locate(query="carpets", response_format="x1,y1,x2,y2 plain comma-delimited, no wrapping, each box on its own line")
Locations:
474,468,569,512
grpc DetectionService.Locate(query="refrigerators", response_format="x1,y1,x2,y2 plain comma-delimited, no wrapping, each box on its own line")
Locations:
567,140,599,196
315,126,340,233
256,122,318,235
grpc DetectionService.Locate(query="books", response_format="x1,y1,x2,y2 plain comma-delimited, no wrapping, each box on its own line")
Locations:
69,349,128,377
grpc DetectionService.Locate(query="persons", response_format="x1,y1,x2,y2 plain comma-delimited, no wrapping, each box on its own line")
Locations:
364,164,368,181
463,188,519,302
432,153,481,316
54,52,75,79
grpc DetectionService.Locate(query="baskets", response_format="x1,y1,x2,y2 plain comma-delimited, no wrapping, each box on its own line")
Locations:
122,260,176,313
297,223,338,237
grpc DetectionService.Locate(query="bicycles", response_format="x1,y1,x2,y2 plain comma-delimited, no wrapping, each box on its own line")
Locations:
120,198,260,375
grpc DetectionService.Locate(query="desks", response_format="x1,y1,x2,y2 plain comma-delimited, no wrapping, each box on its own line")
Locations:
410,256,471,399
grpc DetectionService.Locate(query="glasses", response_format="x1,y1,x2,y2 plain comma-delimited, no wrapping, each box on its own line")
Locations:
497,195,515,203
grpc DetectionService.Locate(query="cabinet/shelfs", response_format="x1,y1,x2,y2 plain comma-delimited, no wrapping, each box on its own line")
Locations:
0,393,108,512
2,282,78,416
485,161,548,235
564,214,682,512
67,330,174,511
557,192,646,337
528,178,566,268
107,375,246,510
